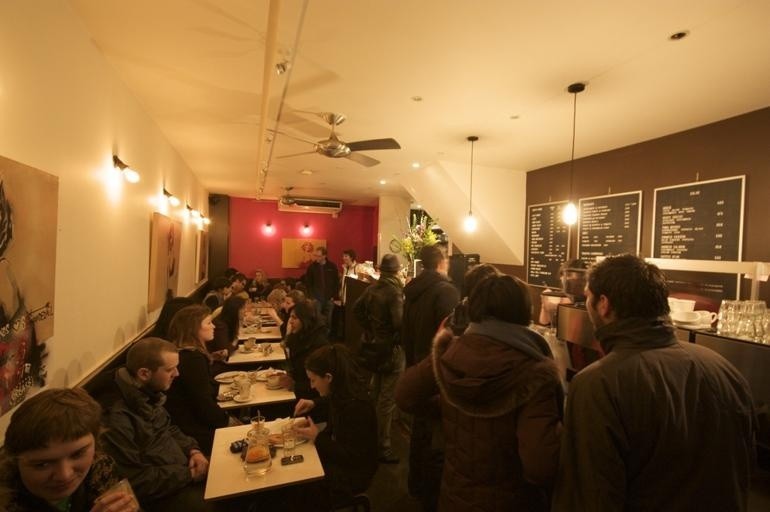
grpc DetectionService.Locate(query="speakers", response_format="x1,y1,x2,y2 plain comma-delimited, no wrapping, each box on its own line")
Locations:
452,254,480,281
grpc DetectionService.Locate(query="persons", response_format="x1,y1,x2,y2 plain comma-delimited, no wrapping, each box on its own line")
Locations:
100,338,257,511
549,254,761,512
1,387,138,511
201,267,272,356
272,246,564,511
168,304,244,450
156,297,227,361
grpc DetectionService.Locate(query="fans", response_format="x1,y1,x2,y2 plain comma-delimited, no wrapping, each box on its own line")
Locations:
266,113,401,166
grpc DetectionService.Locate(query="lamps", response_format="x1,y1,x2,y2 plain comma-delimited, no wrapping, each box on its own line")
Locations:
200,214,209,224
562,83,585,225
462,136,479,232
188,204,201,220
304,224,311,234
114,156,140,183
163,188,180,207
264,223,272,233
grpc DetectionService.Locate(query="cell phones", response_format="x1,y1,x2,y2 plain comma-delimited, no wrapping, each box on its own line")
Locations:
281,455,303,464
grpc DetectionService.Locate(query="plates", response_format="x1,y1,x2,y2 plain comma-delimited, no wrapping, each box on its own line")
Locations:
233,394,254,402
214,371,242,384
274,438,306,448
256,369,287,382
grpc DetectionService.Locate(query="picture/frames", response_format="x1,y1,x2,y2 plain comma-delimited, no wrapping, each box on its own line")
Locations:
195,228,207,284
148,212,182,314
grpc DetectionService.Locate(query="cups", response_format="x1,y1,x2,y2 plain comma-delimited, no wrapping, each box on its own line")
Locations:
282,429,296,457
268,376,279,387
250,416,265,428
240,378,250,399
668,298,770,344
94,478,142,512
238,337,256,352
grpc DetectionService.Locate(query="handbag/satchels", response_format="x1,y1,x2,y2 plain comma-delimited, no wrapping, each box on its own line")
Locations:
361,332,400,376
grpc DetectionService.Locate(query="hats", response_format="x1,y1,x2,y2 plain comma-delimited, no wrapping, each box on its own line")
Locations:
375,254,404,272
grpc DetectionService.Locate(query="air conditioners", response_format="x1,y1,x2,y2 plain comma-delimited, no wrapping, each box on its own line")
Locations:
278,197,343,214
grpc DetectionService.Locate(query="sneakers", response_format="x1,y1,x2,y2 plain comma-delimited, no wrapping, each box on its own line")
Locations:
385,453,400,465
